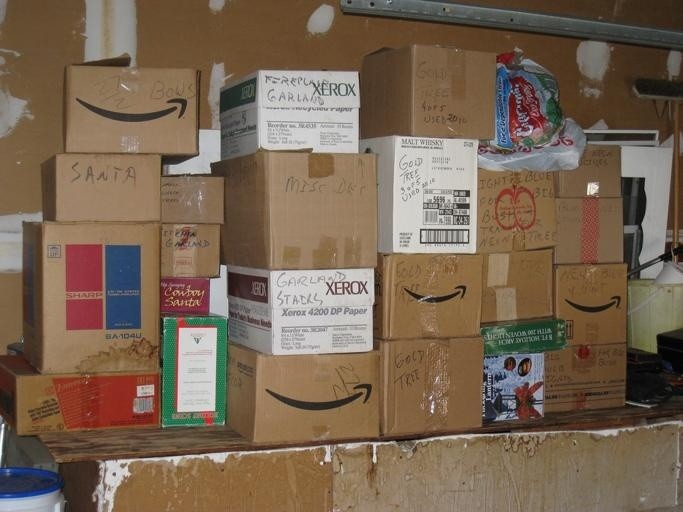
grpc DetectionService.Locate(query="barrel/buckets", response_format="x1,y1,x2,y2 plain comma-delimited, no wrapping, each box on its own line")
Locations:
0,467,69,512
